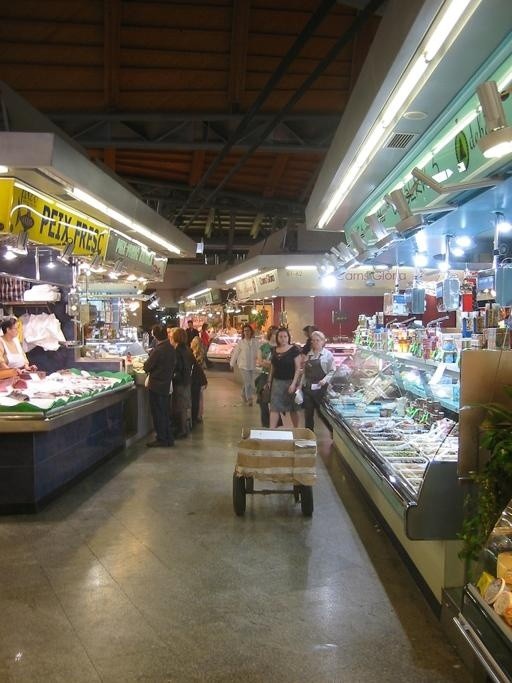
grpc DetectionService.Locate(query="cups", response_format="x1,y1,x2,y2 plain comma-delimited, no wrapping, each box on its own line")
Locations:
452,386,459,401
397,340,411,352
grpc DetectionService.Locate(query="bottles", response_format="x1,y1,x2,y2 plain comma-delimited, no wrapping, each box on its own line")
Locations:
95,329,100,338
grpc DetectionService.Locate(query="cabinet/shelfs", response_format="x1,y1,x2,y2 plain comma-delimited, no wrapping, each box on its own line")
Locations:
439,500,512,681
320,344,507,611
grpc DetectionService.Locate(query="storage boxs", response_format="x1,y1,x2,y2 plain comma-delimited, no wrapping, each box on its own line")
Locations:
236,426,317,485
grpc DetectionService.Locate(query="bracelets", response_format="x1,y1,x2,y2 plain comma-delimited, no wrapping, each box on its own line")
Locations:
267,380,272,383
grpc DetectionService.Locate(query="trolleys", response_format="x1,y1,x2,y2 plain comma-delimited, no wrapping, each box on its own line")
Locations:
232,423,319,517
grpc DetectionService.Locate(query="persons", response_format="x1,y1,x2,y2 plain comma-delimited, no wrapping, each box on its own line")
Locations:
295,328,336,440
229,324,258,406
227,322,237,335
262,329,302,426
143,316,214,448
299,325,317,353
253,325,279,428
0,312,40,378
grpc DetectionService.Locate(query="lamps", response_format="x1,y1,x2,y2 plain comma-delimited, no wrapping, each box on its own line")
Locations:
311,2,512,290
177,265,280,325
0,164,190,322
